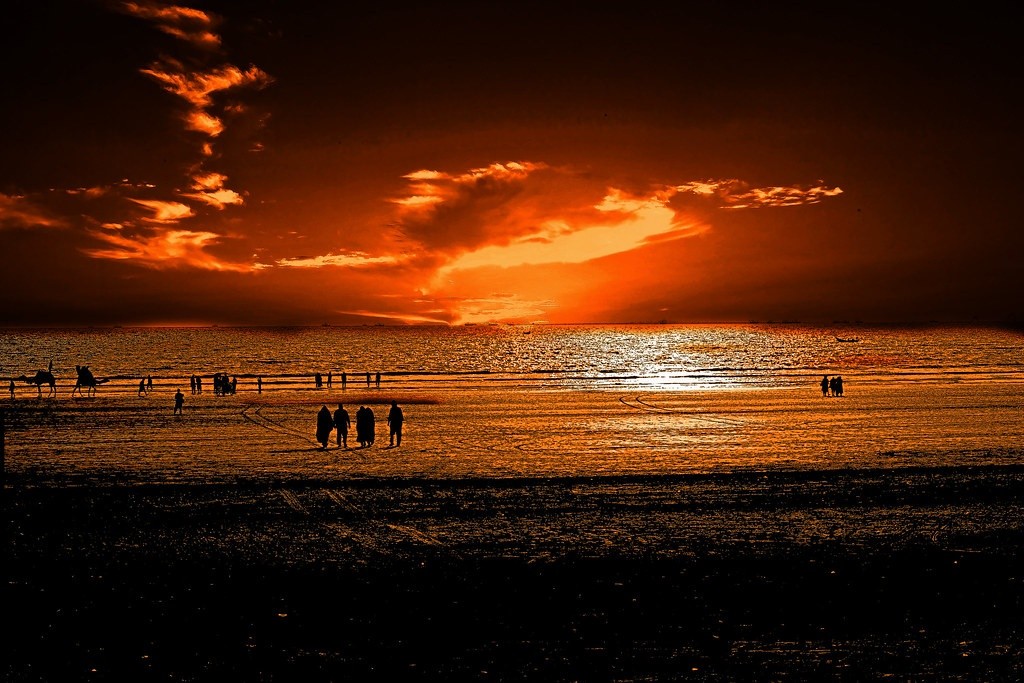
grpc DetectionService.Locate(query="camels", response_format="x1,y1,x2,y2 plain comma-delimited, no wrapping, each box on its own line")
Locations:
72,365,110,398
18,370,57,400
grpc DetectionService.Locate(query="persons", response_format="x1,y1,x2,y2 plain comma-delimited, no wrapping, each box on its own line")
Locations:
9,371,382,415
820,375,843,397
316,405,335,448
356,406,375,448
386,400,404,447
333,404,351,448
